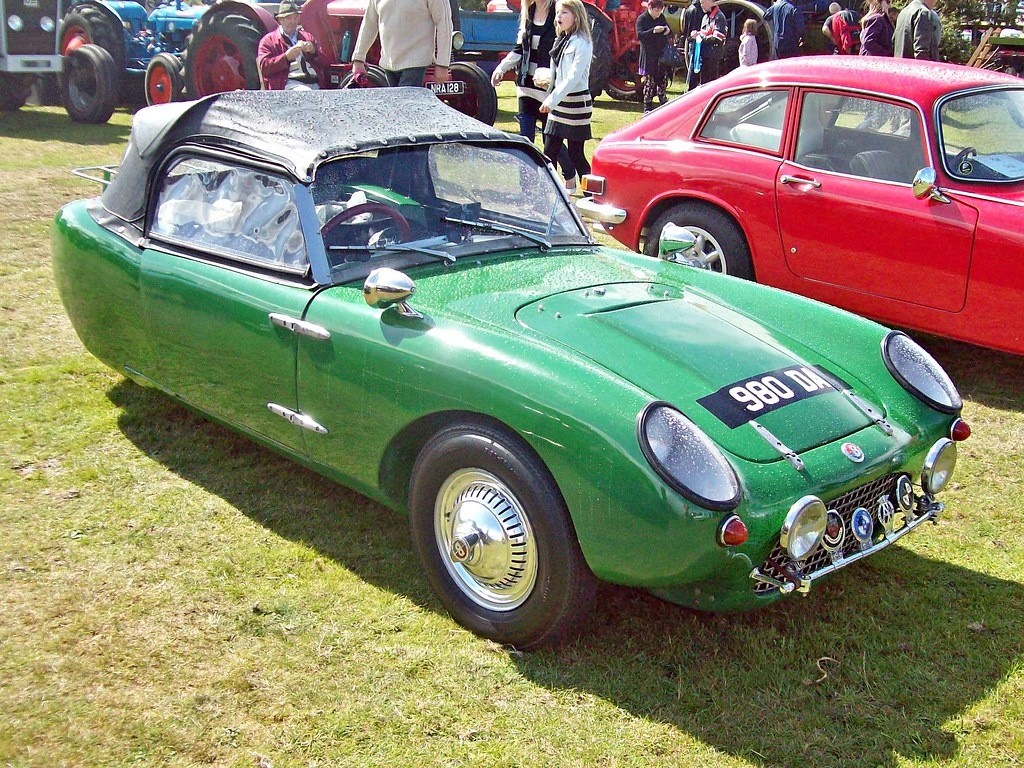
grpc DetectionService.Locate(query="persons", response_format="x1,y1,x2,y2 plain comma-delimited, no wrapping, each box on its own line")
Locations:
490,1,593,198
636,0,941,114
351,1,453,88
258,0,331,90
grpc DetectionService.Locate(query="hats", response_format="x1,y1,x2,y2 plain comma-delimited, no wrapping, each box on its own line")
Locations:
276,0,303,18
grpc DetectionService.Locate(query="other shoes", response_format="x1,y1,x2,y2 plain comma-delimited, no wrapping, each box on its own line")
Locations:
644,108,653,114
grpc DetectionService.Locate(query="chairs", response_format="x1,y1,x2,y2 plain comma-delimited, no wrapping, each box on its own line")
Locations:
849,150,902,181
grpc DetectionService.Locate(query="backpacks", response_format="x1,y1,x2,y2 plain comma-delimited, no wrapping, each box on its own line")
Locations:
832,9,862,55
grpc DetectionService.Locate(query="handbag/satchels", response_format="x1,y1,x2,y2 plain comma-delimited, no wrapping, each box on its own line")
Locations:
694,36,702,73
657,36,685,72
339,61,395,89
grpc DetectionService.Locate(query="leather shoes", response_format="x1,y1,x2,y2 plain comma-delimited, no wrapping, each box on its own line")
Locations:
565,177,577,195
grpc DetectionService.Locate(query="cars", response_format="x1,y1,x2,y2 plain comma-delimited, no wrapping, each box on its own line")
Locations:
48,84,974,655
581,54,1024,357
